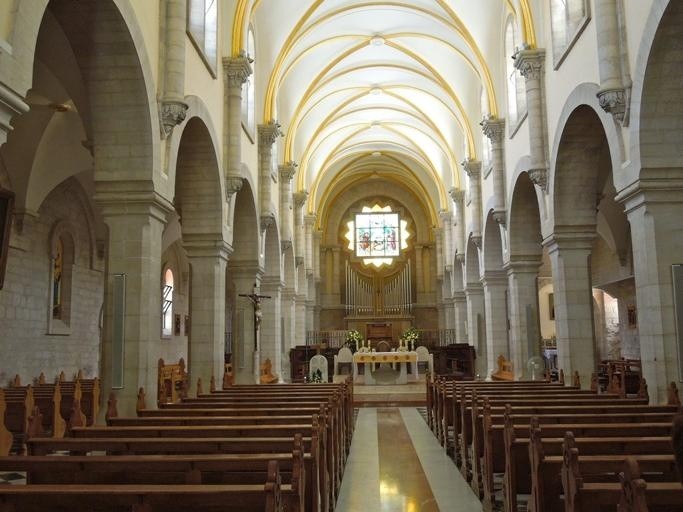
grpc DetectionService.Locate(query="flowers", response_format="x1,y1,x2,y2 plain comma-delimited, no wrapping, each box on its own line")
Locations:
344,328,363,344
403,327,419,342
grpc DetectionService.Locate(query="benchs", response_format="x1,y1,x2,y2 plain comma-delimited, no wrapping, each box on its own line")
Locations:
426,368,682,512
1,379,353,512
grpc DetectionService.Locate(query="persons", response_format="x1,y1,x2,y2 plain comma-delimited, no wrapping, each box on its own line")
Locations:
247,294,263,332
